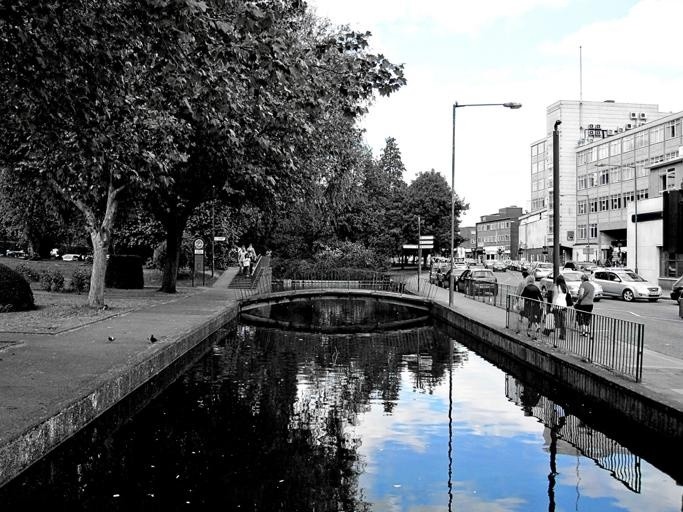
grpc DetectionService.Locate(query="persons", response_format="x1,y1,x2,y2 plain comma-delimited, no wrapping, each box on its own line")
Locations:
237,243,272,277
553,403,565,425
521,385,540,415
518,272,594,340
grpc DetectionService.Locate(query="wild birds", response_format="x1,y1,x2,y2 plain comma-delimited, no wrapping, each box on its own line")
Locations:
106,335,117,343
150,334,157,343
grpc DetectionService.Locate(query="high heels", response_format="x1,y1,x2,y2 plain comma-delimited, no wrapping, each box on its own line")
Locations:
518,296,525,312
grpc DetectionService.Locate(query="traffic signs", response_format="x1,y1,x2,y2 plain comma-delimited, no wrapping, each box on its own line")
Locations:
419,245,434,249
213,236,226,242
419,235,435,239
401,244,418,249
419,241,434,244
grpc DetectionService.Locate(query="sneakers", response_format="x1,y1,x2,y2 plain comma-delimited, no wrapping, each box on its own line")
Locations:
535,326,540,333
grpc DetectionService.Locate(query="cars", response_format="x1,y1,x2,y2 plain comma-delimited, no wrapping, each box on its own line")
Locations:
441,267,466,292
61,253,86,261
588,269,663,303
483,258,554,282
428,255,476,285
539,270,603,303
669,273,682,304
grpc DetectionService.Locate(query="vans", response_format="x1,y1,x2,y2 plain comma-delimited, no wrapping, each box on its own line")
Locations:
6,251,39,260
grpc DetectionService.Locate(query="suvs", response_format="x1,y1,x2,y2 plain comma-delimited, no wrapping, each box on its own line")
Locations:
456,269,497,297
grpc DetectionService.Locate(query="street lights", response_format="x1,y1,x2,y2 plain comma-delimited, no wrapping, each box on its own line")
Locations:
449,102,522,310
593,163,638,275
559,194,590,262
509,218,527,259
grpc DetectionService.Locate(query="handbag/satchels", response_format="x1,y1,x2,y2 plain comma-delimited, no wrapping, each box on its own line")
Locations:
574,298,583,309
566,286,573,306
545,307,555,330
